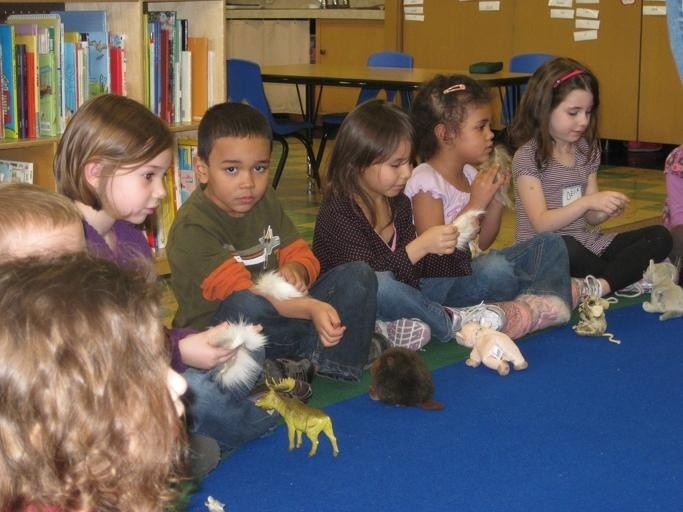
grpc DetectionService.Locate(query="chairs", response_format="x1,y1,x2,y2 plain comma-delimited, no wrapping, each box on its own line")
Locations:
498,53,556,132
311,48,418,175
224,55,325,194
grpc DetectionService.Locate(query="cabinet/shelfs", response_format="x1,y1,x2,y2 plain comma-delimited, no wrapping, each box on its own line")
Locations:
399,1,683,151
0,1,227,285
224,9,388,120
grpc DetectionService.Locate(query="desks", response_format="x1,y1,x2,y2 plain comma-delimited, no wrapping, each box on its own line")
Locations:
252,60,538,166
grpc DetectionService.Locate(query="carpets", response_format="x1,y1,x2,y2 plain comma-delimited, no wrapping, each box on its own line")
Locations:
187,295,681,512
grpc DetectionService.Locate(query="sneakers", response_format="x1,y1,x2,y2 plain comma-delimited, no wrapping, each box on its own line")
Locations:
374,316,433,352
619,260,680,295
249,331,391,420
568,274,603,312
441,303,507,341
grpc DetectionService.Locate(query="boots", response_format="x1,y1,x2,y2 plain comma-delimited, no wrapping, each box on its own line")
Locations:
487,292,574,342
